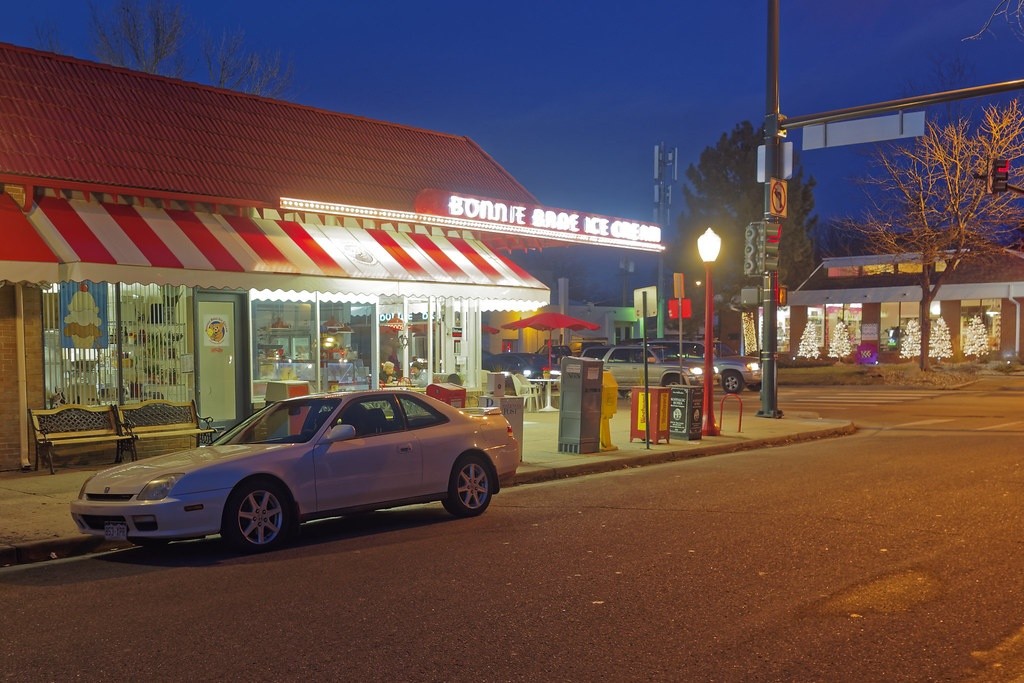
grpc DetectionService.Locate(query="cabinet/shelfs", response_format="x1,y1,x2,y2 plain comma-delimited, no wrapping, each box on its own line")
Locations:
257,358,370,394
267,332,313,358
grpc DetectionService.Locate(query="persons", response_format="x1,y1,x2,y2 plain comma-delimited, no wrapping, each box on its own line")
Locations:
378,361,399,383
407,361,429,387
884,326,899,338
837,317,855,340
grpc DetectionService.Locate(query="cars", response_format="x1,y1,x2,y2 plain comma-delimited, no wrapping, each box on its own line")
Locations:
483,336,763,396
70,387,521,549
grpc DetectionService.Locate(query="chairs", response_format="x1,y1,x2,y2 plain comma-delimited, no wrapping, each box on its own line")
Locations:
511,374,544,410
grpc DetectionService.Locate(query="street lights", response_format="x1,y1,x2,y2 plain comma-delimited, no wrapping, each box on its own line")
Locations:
696,228,722,436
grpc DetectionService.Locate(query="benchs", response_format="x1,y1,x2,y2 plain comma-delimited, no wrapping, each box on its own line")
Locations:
116,397,219,461
28,404,134,476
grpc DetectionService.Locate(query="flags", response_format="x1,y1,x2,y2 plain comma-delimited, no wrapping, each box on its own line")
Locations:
668,300,679,319
681,298,692,319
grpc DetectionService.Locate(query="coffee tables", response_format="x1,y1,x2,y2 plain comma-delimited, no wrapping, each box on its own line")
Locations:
527,378,561,412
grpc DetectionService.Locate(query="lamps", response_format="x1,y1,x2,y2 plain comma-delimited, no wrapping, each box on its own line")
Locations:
322,302,344,328
268,300,291,330
387,282,404,324
336,301,355,333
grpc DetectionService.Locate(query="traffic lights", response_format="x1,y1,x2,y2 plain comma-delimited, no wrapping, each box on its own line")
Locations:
985,158,1009,194
763,220,780,272
777,286,786,307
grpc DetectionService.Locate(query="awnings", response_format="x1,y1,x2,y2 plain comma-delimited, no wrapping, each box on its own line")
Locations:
0,191,550,312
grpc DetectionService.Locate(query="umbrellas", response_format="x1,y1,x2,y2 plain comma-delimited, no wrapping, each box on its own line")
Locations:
501,312,602,371
